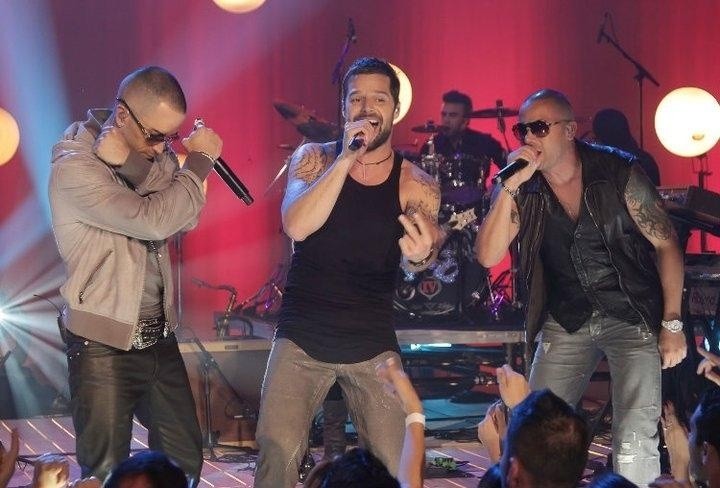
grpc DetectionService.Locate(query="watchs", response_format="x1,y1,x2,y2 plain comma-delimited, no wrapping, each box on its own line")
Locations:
660,317,685,336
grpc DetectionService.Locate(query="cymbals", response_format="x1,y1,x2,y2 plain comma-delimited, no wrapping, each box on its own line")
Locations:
411,120,450,135
462,101,519,117
273,100,344,141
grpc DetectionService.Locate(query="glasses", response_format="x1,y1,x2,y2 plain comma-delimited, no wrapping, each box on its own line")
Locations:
112,92,183,148
510,117,574,142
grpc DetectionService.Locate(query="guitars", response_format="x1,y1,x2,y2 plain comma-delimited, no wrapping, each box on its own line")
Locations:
439,205,482,238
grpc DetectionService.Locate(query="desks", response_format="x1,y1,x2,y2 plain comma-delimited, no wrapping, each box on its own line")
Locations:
178,310,526,464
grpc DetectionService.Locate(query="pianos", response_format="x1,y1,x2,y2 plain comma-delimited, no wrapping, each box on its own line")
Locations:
656,185,720,238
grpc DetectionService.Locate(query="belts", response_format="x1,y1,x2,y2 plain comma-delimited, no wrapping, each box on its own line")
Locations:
118,313,178,351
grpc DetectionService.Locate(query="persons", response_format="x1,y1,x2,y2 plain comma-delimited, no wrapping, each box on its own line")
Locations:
417,89,512,325
294,355,426,488
43,64,224,488
251,54,442,487
472,86,686,488
688,314,720,486
474,362,689,488
593,106,662,190
0,422,195,488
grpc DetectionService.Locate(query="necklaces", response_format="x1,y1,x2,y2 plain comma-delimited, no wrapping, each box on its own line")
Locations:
355,151,393,167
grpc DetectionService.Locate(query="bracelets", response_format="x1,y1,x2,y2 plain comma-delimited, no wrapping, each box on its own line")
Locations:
407,246,435,267
402,411,427,431
501,182,522,198
199,151,217,166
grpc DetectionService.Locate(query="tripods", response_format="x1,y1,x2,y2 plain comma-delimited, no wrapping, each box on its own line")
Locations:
191,335,256,462
233,136,309,320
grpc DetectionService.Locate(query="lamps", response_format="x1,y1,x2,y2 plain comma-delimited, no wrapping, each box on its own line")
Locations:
653,87,720,253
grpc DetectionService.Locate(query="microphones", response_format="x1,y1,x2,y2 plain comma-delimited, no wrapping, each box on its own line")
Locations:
492,152,535,188
596,17,607,43
190,128,255,208
347,128,373,152
348,17,358,44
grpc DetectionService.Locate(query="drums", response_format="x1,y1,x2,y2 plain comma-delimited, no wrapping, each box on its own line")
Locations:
430,153,487,188
391,212,484,318
